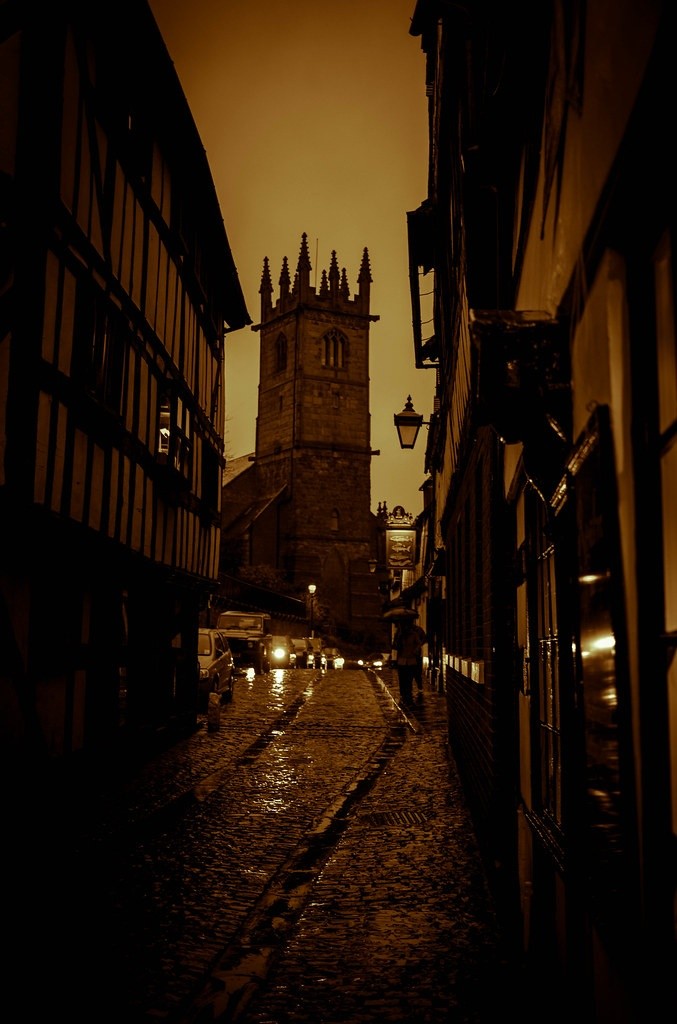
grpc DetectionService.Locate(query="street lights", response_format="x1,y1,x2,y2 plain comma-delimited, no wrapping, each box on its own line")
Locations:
308,584,317,638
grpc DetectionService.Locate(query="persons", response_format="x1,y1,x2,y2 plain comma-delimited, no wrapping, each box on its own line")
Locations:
392,617,427,703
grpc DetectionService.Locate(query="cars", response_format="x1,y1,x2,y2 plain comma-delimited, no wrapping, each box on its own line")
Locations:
171,628,236,702
272,634,392,670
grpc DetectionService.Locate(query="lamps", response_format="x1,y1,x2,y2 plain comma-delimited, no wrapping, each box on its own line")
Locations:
394,394,446,450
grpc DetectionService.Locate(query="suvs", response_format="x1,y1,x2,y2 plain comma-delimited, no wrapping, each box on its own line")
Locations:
215,610,274,675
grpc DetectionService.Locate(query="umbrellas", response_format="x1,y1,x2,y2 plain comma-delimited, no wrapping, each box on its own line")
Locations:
383,609,420,618
